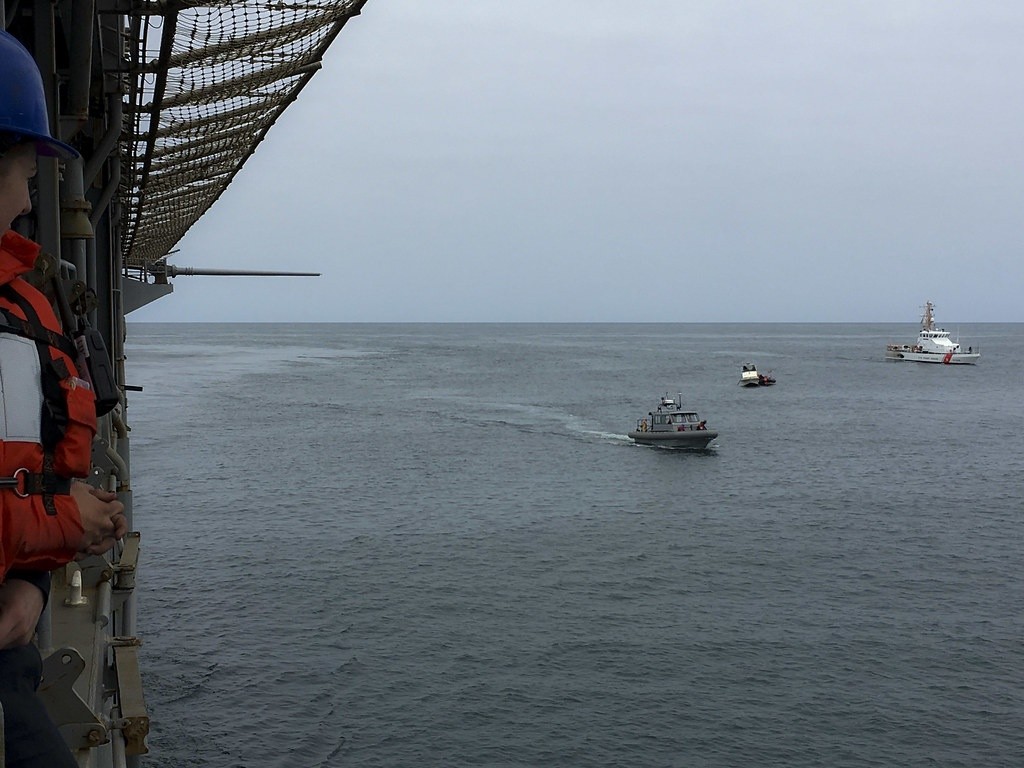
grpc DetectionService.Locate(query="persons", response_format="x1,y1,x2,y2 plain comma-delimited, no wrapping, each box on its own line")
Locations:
0,30,127,768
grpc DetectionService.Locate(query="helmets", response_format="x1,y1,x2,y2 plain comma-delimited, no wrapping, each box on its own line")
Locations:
0,29,79,161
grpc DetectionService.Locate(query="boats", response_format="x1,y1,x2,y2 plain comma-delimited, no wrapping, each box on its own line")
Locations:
885,300,981,364
736,362,777,387
627,391,719,451
